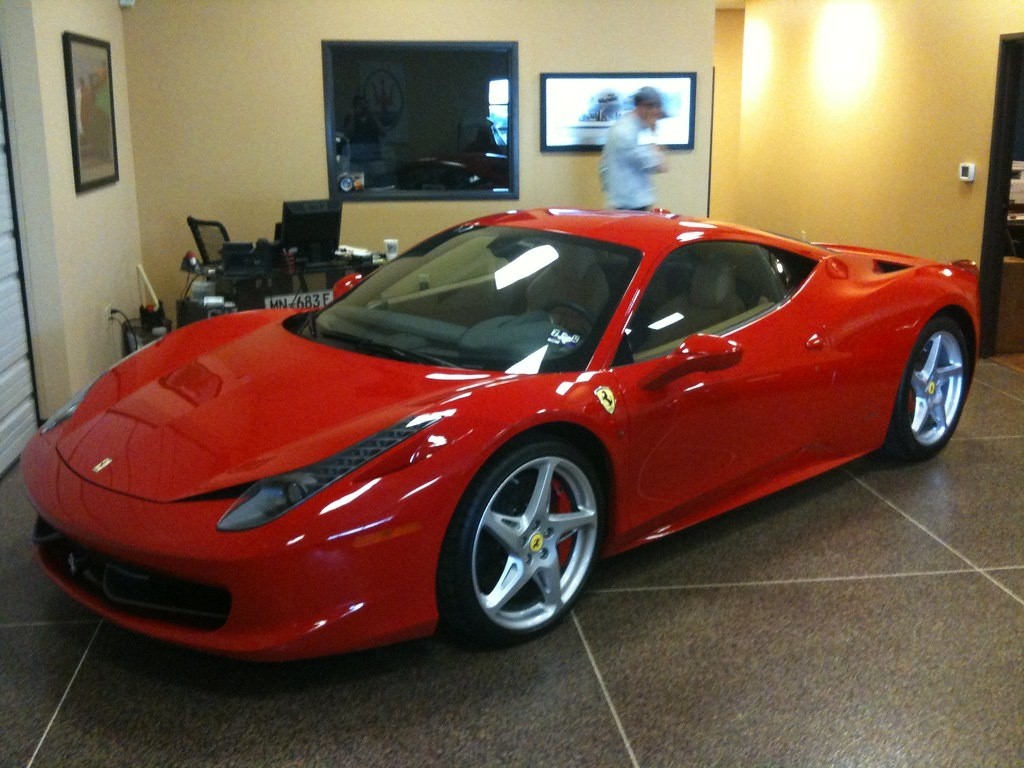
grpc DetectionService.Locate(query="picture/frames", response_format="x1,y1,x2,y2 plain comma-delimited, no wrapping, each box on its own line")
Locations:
62,31,121,198
539,72,697,151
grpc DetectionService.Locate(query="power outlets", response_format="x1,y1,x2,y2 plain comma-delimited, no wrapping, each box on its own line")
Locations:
104,302,114,327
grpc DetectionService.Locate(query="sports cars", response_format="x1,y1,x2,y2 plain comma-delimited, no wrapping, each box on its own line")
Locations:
19,209,982,664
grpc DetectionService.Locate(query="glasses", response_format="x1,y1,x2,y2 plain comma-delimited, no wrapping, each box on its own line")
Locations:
638,102,661,109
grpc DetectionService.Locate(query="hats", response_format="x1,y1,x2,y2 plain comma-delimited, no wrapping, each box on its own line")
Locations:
633,86,658,106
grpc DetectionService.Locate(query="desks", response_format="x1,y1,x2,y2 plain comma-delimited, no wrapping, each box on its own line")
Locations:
183,255,401,312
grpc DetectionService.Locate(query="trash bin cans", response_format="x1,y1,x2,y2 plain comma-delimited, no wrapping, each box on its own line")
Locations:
123,317,173,356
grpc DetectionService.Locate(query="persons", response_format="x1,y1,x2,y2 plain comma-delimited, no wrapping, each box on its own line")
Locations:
598,86,671,210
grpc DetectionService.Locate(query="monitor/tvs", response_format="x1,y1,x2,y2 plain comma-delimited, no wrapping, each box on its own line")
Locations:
281,199,343,264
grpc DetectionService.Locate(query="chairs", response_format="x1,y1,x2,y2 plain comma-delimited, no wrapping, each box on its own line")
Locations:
187,215,231,281
632,263,748,354
526,243,612,327
